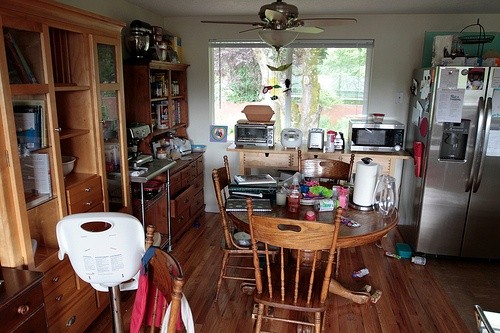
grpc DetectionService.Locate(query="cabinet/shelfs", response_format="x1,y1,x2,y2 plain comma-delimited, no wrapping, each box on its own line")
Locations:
225,142,411,177
0,0,206,333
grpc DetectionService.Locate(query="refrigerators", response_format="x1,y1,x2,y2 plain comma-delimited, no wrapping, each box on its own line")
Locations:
396,65,500,259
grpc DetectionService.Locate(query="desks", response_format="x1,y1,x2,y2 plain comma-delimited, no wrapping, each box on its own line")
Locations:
225,180,400,305
128,160,177,253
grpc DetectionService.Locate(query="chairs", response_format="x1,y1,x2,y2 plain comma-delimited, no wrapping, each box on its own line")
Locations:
55,211,160,333
212,149,355,333
129,224,185,333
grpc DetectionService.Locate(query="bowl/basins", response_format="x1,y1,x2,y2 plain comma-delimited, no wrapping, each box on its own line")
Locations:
60,155,76,175
125,35,149,58
234,232,258,246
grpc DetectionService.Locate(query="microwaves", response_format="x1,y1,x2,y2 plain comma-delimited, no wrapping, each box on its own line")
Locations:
348,118,404,153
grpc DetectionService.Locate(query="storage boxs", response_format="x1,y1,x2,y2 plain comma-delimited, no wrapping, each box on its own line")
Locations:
395,241,412,259
167,33,184,63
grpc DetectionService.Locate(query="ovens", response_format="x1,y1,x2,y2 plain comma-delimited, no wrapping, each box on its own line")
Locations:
234,124,275,148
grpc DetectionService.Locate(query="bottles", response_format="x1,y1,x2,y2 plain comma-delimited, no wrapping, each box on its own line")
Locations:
373,175,397,218
411,256,426,265
288,187,300,212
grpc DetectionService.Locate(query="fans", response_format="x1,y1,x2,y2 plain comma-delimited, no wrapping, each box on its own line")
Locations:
200,0,358,51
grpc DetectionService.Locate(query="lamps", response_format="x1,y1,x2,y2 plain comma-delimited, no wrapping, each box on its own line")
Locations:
255,22,298,51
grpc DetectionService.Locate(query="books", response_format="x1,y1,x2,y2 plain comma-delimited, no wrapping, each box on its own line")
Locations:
157,102,181,129
13,99,47,150
228,174,276,194
4,32,37,84
150,76,178,98
226,199,271,211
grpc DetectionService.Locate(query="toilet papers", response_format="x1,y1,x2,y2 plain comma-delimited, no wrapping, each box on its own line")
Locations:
353,161,382,207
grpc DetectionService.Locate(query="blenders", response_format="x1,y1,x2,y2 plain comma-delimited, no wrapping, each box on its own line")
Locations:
125,19,154,64
